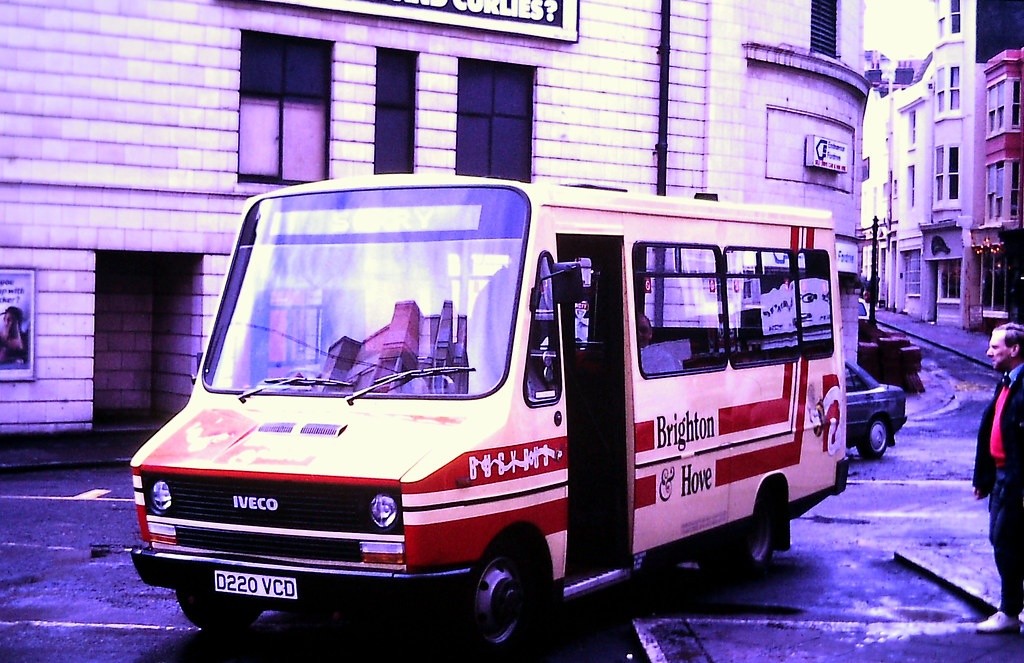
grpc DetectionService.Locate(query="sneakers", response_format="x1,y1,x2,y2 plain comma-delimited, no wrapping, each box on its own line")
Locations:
976,611,1019,633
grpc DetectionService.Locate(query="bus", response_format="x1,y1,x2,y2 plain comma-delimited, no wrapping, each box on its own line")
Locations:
88,172,851,663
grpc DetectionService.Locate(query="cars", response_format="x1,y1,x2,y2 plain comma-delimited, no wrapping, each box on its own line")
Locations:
843,359,908,461
858,298,870,323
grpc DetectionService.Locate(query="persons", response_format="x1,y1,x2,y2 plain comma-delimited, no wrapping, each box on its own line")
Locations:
0,306,29,365
635,312,684,374
971,323,1024,633
468,239,521,394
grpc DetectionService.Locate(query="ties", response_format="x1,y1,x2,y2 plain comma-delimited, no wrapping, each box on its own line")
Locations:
1004,375,1011,389
